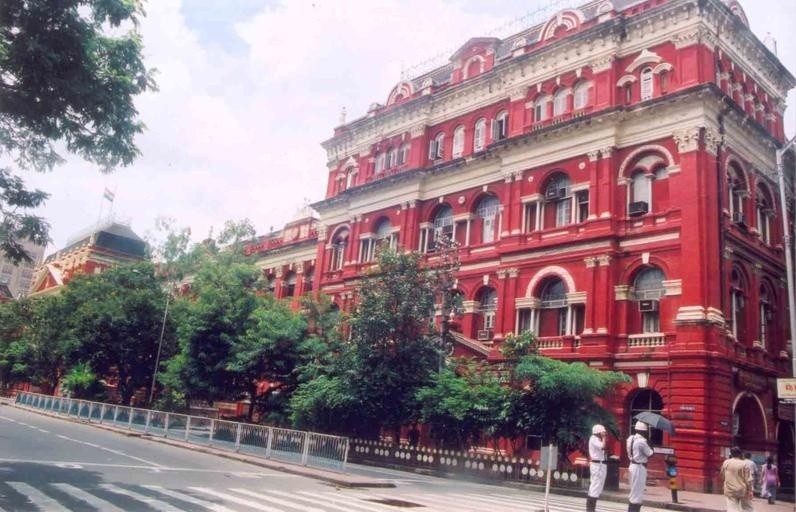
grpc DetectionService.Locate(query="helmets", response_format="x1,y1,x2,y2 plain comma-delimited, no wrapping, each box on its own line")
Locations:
634,421,648,431
592,424,606,435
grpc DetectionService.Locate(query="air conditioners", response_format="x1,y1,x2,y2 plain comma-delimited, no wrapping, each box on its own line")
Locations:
638,299,658,313
546,187,563,199
733,211,747,227
629,201,648,215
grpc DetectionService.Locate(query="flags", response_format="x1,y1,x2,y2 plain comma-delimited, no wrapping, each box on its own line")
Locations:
103,187,115,202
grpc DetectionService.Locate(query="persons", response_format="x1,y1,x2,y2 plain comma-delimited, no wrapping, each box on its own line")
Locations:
406,422,420,446
742,452,758,490
760,455,782,505
586,424,607,511
760,460,768,498
626,420,655,512
719,446,754,511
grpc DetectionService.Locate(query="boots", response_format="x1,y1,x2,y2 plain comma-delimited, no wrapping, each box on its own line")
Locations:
628,502,641,512
586,496,597,512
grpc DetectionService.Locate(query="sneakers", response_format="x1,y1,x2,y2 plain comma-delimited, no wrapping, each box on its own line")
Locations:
768,496,775,504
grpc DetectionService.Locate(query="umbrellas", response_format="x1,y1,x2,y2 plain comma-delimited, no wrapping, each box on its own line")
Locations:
633,411,677,437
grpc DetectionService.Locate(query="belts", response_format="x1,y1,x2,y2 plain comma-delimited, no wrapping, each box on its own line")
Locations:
631,461,647,467
590,460,607,464
